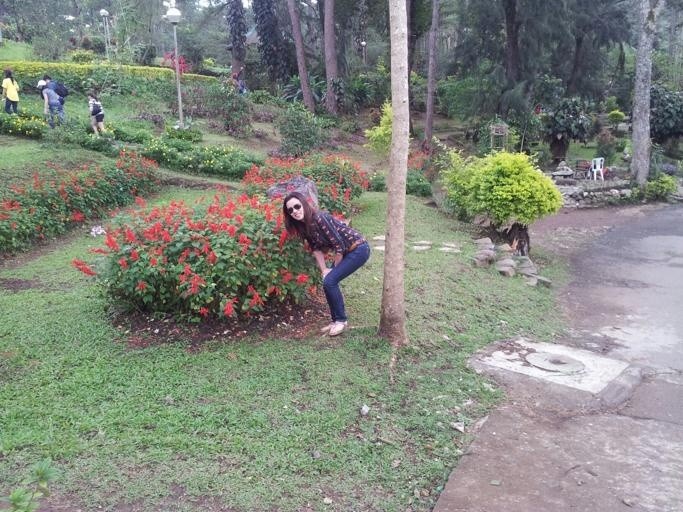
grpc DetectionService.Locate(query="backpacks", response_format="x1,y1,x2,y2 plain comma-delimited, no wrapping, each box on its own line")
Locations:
54,84,68,97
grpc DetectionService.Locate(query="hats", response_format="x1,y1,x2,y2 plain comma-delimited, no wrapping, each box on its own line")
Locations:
36,80,46,88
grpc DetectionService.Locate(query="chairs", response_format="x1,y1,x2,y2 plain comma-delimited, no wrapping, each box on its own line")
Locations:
556,158,604,182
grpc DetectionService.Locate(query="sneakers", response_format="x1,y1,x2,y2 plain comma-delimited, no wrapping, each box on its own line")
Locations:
319,322,334,332
329,321,347,336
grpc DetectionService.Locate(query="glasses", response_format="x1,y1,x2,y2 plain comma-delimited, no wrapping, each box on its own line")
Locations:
285,204,301,214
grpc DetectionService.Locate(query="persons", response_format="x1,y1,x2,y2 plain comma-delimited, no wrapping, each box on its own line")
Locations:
86,92,104,136
1,69,19,114
42,75,64,125
231,66,245,94
35,80,63,129
282,190,371,337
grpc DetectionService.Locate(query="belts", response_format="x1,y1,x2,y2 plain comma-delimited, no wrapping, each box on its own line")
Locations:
348,239,365,252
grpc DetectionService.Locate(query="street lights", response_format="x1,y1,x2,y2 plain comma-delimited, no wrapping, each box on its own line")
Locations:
164,6,184,132
361,40,368,64
98,8,109,58
105,9,111,46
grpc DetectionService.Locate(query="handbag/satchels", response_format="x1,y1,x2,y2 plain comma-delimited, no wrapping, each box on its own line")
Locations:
92,105,101,115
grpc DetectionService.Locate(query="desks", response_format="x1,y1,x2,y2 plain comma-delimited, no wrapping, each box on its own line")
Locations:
552,171,573,179
